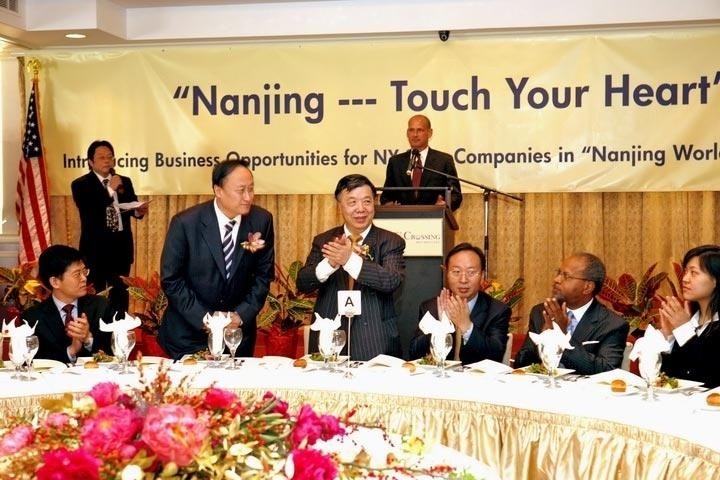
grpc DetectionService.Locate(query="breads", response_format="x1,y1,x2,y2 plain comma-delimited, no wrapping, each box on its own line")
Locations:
513,370,524,374
294,359,307,369
612,380,626,392
402,363,415,373
84,361,99,369
706,393,720,406
183,359,196,365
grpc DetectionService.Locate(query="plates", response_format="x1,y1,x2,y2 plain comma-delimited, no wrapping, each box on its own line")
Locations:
599,384,640,397
0,359,55,375
75,354,118,369
405,354,463,370
513,362,577,378
285,357,317,374
638,375,707,393
392,363,425,377
176,352,230,367
64,364,110,377
169,360,209,375
496,369,538,386
695,386,720,411
297,350,351,364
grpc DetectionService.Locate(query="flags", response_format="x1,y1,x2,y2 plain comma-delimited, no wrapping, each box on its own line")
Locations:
13,67,53,266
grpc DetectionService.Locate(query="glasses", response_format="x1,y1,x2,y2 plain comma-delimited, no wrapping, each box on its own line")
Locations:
552,268,594,283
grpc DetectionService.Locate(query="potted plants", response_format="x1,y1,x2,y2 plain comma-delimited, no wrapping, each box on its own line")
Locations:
120,270,168,355
484,260,685,377
256,260,313,356
0,255,51,362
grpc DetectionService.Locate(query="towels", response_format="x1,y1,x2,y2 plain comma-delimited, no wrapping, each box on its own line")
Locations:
202,310,233,357
419,310,456,362
99,312,142,360
1,317,39,366
311,312,342,358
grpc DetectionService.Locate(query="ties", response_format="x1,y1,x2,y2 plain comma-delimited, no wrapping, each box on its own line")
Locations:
102,178,121,233
346,233,363,290
221,219,236,280
453,328,463,361
412,153,423,198
566,310,575,332
61,304,77,326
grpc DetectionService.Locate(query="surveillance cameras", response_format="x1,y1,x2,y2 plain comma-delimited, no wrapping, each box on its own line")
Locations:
439,31,449,41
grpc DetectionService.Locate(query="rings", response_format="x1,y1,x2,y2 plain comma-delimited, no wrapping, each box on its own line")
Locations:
550,317,556,321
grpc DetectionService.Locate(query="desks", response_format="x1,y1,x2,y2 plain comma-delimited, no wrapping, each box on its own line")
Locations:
0,353,720,480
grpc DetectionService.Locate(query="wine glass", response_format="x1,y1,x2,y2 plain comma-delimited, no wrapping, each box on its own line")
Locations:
536,339,562,391
431,332,452,379
319,329,346,365
108,329,139,375
639,350,664,403
207,328,243,373
7,335,40,382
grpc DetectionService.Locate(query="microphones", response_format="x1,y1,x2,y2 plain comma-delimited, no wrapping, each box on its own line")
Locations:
406,149,419,176
109,167,125,193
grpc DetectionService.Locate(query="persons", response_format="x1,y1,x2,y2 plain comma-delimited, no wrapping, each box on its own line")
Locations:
380,113,464,211
296,173,409,360
657,244,719,389
512,252,630,373
70,139,148,317
158,157,278,359
17,242,119,365
409,240,513,362
